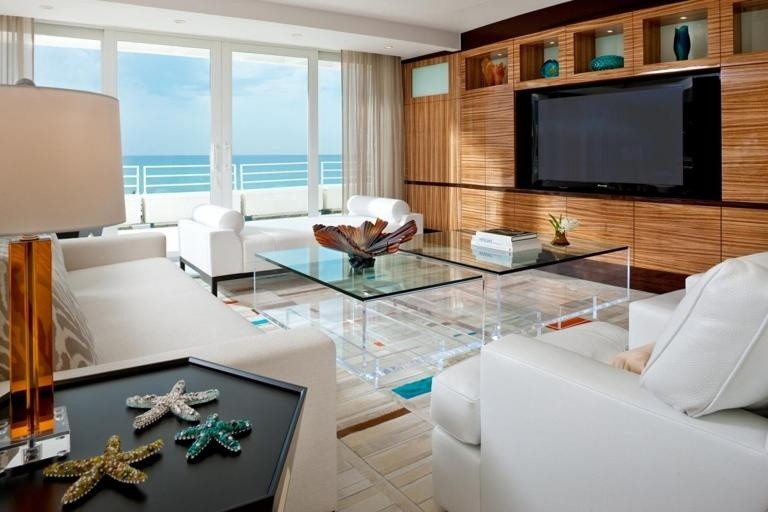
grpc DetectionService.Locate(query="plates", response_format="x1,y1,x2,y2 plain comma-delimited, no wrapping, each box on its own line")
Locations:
539,58,559,78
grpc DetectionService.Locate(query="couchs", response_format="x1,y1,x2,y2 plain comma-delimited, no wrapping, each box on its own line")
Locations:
177,191,424,293
431,253,768,512
0,233,341,512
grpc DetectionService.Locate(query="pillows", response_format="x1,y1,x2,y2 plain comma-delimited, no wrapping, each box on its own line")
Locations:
346,196,409,225
193,204,245,233
639,252,768,420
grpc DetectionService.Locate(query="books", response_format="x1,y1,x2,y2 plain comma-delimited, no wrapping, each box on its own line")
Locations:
471,245,538,268
470,227,541,253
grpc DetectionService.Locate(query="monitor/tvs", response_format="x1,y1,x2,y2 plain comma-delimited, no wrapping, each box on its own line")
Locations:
532,76,695,195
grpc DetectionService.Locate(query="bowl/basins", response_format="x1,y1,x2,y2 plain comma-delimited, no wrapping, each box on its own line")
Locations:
591,55,625,70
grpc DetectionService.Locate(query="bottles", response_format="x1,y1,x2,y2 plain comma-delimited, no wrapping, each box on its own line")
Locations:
674,25,690,61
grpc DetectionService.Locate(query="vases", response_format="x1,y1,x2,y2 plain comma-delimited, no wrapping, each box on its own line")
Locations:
550,229,570,246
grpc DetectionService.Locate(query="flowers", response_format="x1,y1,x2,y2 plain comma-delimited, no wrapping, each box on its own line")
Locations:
549,213,581,234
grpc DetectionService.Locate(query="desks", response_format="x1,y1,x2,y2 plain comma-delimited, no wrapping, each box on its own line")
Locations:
0,349,307,511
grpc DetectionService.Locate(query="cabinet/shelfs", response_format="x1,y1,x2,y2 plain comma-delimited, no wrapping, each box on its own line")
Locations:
459,0,767,95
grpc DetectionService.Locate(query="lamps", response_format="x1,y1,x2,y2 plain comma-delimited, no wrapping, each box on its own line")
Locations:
0,79,126,475
